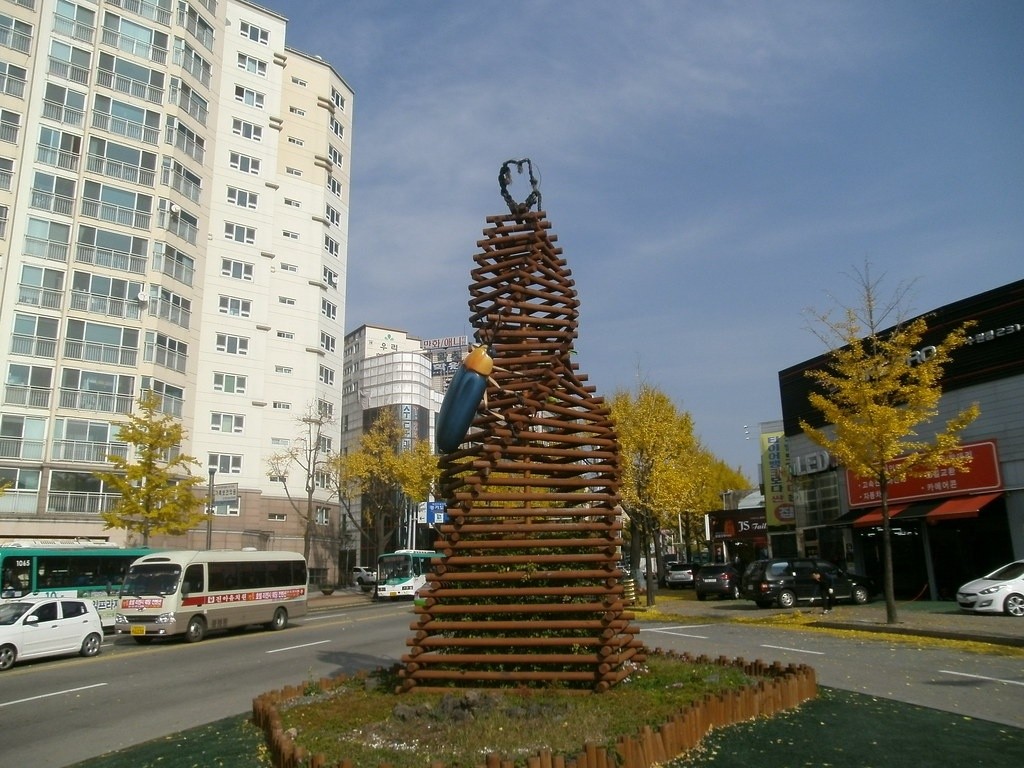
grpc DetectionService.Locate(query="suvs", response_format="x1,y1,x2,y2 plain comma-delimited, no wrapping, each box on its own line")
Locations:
693,560,743,601
667,560,694,590
740,557,878,610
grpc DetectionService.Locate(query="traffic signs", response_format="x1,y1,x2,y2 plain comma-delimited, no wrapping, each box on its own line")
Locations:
418,501,448,524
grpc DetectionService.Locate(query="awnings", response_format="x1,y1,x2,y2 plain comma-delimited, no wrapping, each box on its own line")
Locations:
826,493,1002,529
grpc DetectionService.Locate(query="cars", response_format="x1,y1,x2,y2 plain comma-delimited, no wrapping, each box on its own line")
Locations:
353,567,378,586
956,560,1024,617
0,596,104,672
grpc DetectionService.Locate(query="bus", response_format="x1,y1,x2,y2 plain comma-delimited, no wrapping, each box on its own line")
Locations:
376,549,436,602
0,540,166,628
105,547,310,646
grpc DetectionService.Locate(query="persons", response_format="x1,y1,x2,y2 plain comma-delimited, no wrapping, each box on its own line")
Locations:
810,571,835,614
